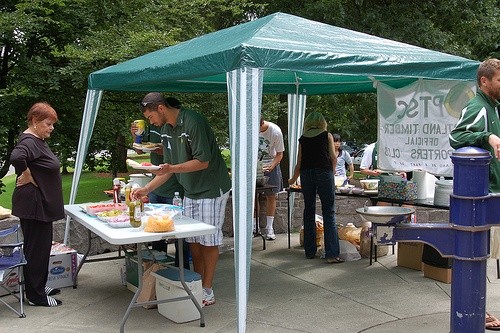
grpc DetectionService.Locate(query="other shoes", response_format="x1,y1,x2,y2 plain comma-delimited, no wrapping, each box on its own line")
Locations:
201,288,215,305
45,286,61,296
28,295,62,307
253,227,262,236
265,226,276,240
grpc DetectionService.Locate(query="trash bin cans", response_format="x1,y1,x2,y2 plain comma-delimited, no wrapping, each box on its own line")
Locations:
356,219,389,257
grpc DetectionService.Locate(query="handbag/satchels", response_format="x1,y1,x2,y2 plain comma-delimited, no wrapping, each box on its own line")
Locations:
135,262,168,309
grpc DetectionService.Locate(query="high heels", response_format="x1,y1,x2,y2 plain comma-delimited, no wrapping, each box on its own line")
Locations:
325,256,345,263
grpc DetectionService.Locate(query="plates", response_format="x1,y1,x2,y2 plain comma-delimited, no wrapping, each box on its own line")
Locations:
134,146,159,151
97,210,108,220
130,165,164,170
435,180,453,188
107,216,131,228
365,190,378,194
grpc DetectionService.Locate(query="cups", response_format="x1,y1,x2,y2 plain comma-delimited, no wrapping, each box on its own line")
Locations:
134,120,145,136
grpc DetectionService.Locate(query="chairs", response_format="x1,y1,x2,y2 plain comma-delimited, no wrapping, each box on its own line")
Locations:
0,224,27,318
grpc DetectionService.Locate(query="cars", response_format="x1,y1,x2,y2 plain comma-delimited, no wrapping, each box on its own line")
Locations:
342,146,357,157
347,148,377,171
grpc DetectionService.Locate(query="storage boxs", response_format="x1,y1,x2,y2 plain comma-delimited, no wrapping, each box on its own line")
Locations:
378,180,417,200
47,246,77,289
129,173,153,187
125,252,175,293
0,266,25,297
424,263,451,284
397,241,424,270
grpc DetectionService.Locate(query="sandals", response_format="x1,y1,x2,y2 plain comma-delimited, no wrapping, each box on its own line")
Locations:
485,311,500,330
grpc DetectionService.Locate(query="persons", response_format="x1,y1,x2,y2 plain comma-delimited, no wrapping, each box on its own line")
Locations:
289,111,343,265
135,91,231,307
257,114,284,241
360,141,407,181
334,135,354,187
9,100,67,307
447,57,499,331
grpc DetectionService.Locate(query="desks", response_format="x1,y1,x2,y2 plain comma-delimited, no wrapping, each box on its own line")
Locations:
285,187,450,265
64,202,216,333
104,184,278,256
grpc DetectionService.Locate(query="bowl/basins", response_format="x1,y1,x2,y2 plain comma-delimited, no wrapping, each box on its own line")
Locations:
356,206,415,222
360,179,380,189
339,187,351,192
353,188,364,193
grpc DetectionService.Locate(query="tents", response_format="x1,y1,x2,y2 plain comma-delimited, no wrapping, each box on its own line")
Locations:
65,13,482,333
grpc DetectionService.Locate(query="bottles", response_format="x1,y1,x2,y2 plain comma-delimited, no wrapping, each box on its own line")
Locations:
173,192,183,219
129,200,142,227
113,178,122,204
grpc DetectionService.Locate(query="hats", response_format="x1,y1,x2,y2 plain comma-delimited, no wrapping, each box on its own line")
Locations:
141,92,164,106
